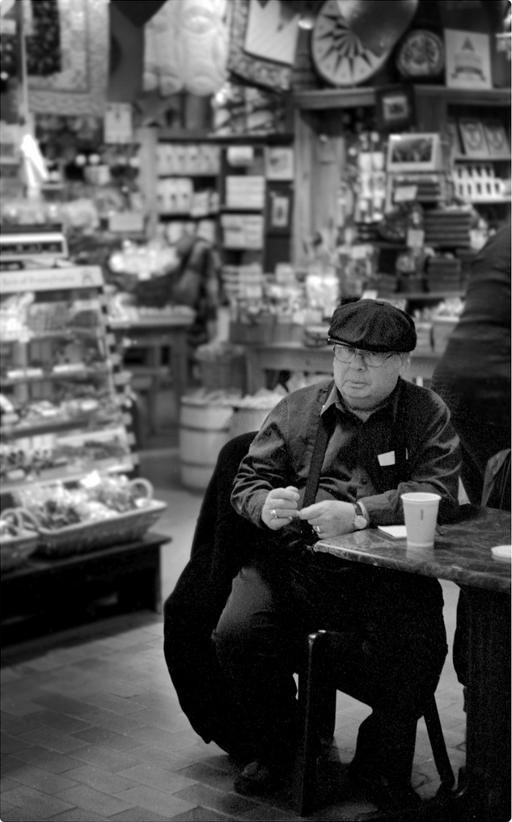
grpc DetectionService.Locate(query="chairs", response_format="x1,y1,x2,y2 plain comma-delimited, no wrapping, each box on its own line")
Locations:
204,430,458,818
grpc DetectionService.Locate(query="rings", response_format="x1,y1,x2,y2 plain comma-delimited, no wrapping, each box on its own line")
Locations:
316,524,321,533
271,508,280,520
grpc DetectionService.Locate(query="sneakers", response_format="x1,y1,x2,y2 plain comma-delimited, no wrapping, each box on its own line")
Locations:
232,740,318,800
360,770,425,813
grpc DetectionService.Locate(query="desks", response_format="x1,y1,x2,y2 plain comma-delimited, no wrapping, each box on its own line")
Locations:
243,342,444,403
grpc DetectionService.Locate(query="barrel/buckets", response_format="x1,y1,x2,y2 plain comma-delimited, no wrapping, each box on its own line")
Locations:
179,397,235,491
196,344,246,396
231,406,276,438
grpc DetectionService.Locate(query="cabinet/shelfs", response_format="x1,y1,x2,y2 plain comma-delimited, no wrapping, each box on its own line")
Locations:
0,266,175,655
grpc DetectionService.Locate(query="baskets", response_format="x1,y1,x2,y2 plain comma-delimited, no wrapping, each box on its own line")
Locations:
20,477,169,559
0,509,40,573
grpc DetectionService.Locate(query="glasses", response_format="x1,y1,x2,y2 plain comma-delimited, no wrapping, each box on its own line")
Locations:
329,342,400,368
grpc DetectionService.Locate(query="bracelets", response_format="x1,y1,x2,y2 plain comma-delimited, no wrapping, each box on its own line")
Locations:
352,501,368,530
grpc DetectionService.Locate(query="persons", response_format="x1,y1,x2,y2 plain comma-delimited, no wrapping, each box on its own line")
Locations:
431,210,510,820
209,296,467,812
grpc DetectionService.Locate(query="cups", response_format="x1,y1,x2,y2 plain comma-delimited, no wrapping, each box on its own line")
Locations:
400,492,442,548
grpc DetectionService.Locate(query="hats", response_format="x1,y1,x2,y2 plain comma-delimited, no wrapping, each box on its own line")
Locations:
326,296,419,353
